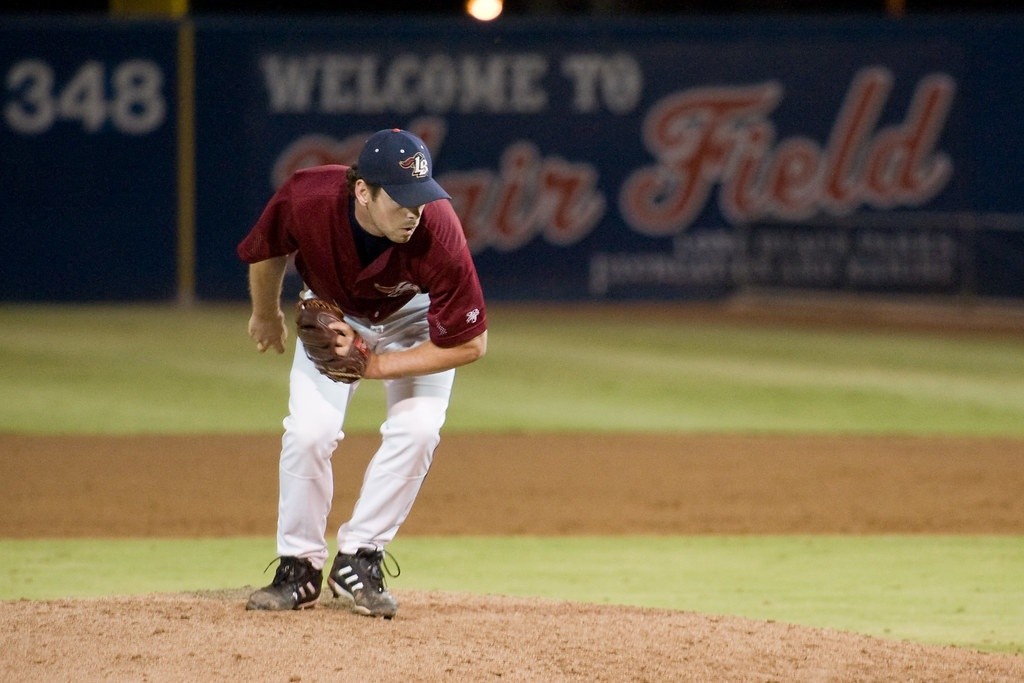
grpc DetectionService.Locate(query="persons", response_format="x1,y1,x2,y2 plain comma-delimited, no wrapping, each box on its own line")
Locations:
237,128,488,616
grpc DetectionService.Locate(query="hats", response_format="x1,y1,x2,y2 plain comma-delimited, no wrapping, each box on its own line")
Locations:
359,129,452,208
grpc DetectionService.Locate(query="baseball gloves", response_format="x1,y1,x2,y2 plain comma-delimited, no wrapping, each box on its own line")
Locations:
295,298,370,385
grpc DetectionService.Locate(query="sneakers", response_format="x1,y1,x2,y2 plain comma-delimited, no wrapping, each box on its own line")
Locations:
246,556,323,610
327,547,401,616
